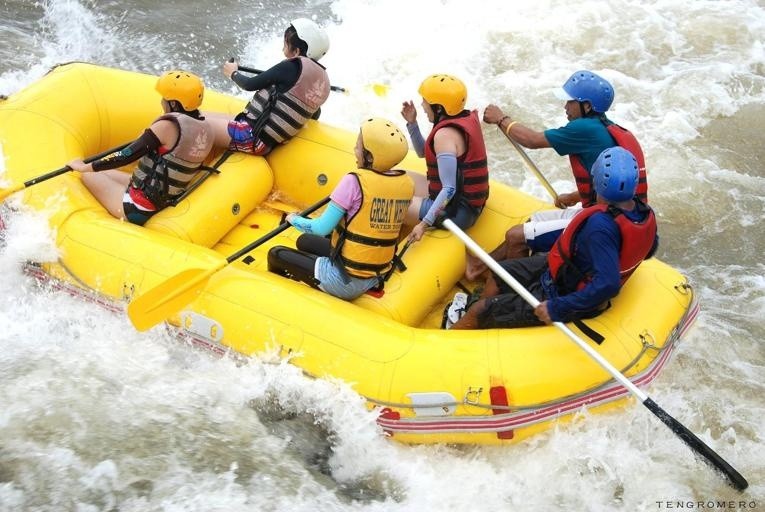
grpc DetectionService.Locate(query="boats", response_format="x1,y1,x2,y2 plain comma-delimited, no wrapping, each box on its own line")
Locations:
0,61,701,447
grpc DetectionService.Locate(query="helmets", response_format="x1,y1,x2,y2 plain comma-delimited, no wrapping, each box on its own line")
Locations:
155,71,204,110
360,117,409,173
591,146,640,202
554,73,616,113
292,18,330,61
417,75,469,116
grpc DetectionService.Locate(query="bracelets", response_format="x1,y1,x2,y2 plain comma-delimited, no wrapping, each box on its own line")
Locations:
505,121,516,134
498,116,509,129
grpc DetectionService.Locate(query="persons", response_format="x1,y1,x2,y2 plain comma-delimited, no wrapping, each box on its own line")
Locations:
445,146,658,330
198,18,330,156
463,70,648,285
268,119,414,300
401,74,489,242
66,72,214,226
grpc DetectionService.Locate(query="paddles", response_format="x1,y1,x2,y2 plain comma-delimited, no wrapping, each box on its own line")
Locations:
0,139,138,205
127,193,332,332
442,219,748,491
228,57,388,97
